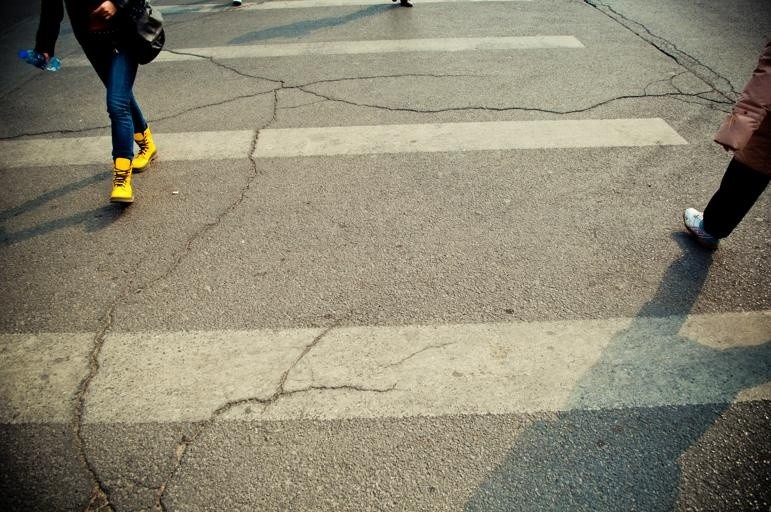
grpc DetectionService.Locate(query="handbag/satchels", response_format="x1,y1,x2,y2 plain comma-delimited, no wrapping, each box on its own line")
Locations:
115,1,165,65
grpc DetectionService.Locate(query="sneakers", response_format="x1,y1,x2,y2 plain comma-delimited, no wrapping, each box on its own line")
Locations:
683,206,712,242
400,0,413,7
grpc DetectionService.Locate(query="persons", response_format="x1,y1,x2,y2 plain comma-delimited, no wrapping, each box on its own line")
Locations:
683,41,770,250
33,0,158,204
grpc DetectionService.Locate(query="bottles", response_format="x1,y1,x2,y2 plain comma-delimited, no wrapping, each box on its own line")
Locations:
15,47,61,73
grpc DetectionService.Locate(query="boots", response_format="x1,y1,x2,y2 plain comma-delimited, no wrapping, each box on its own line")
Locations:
110,157,134,203
132,127,158,172
